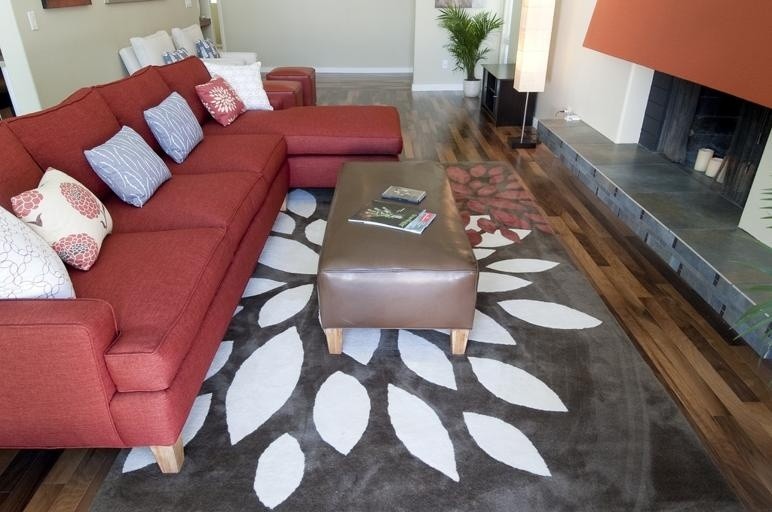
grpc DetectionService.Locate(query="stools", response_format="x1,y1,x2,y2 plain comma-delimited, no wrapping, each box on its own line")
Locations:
266,65,317,106
264,91,296,110
261,79,303,107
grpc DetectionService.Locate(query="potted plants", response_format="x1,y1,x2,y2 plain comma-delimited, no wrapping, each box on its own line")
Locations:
434,2,504,98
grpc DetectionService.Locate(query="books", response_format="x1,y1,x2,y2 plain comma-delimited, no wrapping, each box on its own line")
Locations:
347,199,437,235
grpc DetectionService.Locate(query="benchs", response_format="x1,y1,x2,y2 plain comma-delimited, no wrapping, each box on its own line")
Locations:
316,159,479,355
152,55,404,189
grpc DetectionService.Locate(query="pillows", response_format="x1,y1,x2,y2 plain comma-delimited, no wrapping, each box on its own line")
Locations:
170,22,208,58
193,38,222,59
129,28,175,66
203,61,274,111
161,47,190,65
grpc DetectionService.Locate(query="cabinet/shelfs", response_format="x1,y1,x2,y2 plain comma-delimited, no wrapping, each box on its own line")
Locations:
478,63,537,128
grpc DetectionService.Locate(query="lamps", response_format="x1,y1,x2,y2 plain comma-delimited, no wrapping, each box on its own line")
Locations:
507,0,556,148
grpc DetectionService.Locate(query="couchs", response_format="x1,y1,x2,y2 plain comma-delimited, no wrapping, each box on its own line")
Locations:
0,56,292,476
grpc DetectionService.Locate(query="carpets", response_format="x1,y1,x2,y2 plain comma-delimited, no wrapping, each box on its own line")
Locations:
88,159,747,512
